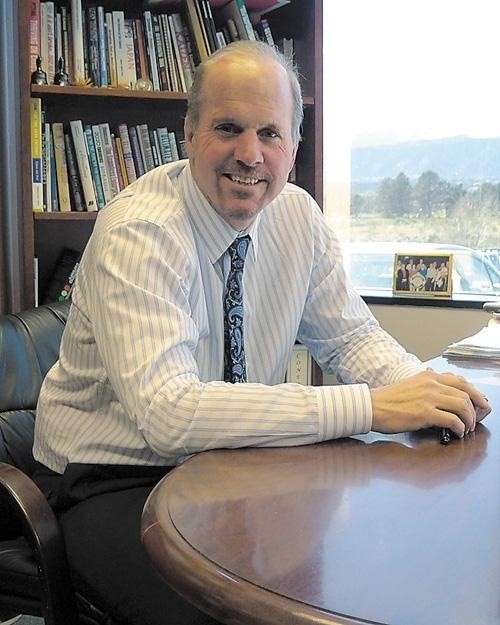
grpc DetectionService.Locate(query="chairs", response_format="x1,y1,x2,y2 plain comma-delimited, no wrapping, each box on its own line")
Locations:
0,300,124,625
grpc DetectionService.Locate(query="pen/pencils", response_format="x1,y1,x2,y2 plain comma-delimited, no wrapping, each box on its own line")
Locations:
441,428,449,445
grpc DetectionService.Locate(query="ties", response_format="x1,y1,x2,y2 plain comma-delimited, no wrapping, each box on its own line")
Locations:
223,237,252,386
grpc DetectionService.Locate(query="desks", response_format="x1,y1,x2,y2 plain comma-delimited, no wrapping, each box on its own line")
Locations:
142,356,500,625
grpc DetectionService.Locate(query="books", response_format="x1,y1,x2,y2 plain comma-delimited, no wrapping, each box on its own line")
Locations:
29,1,292,92
31,98,188,212
34,248,83,309
289,162,296,184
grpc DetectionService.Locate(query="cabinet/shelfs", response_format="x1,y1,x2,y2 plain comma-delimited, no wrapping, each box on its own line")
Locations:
19,0,324,385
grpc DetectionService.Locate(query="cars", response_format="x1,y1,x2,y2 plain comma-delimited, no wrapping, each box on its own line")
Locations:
345,242,500,296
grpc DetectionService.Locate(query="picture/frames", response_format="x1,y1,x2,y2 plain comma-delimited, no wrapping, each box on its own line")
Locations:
392,253,453,297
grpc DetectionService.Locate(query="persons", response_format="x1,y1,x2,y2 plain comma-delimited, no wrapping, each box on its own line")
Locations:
30,39,491,625
395,256,449,292
